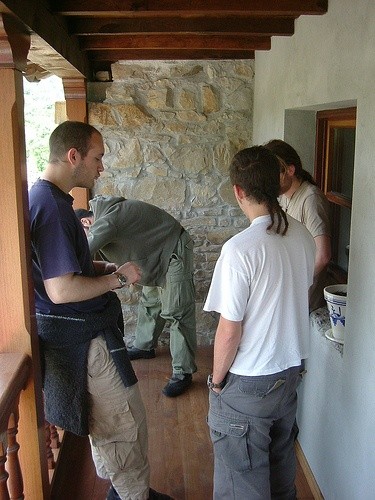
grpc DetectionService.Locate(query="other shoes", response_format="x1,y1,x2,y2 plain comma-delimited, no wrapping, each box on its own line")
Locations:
163,373,193,396
107,483,174,499
126,346,155,360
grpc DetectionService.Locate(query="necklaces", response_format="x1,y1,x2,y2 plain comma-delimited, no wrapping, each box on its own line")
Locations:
285,181,304,212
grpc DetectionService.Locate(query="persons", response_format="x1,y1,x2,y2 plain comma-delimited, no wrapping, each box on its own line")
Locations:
203,146,316,500
266,140,332,311
73,195,197,398
29,120,172,500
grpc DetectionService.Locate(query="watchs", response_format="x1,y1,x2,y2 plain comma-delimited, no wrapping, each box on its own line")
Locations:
207,374,226,389
112,272,127,290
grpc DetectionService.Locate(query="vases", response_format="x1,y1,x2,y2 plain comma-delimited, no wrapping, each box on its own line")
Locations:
323,284,346,341
